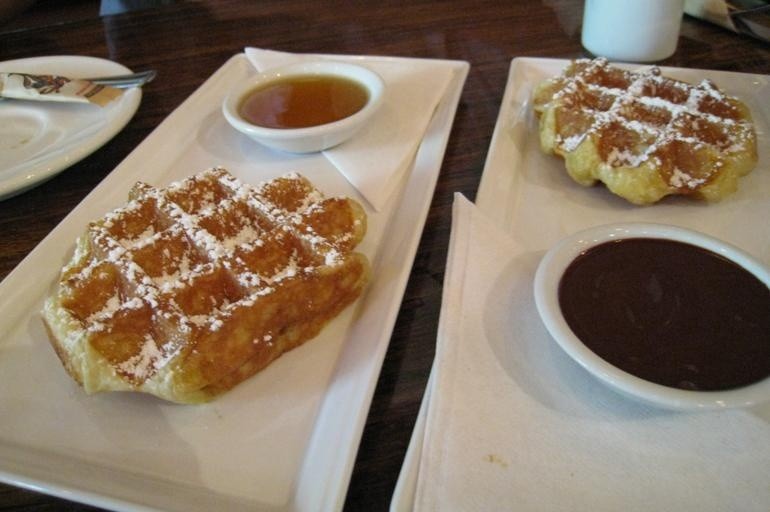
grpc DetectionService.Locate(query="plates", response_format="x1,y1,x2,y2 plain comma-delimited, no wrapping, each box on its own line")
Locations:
222,60,385,155
0,52,471,512
0,54,143,202
532,223,770,413
388,56,770,512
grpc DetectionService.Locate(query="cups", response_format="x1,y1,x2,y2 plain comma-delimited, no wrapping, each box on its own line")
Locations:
580,0,686,63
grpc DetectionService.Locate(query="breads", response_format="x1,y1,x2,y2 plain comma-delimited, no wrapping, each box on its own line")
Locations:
38,164,369,408
532,56,760,206
